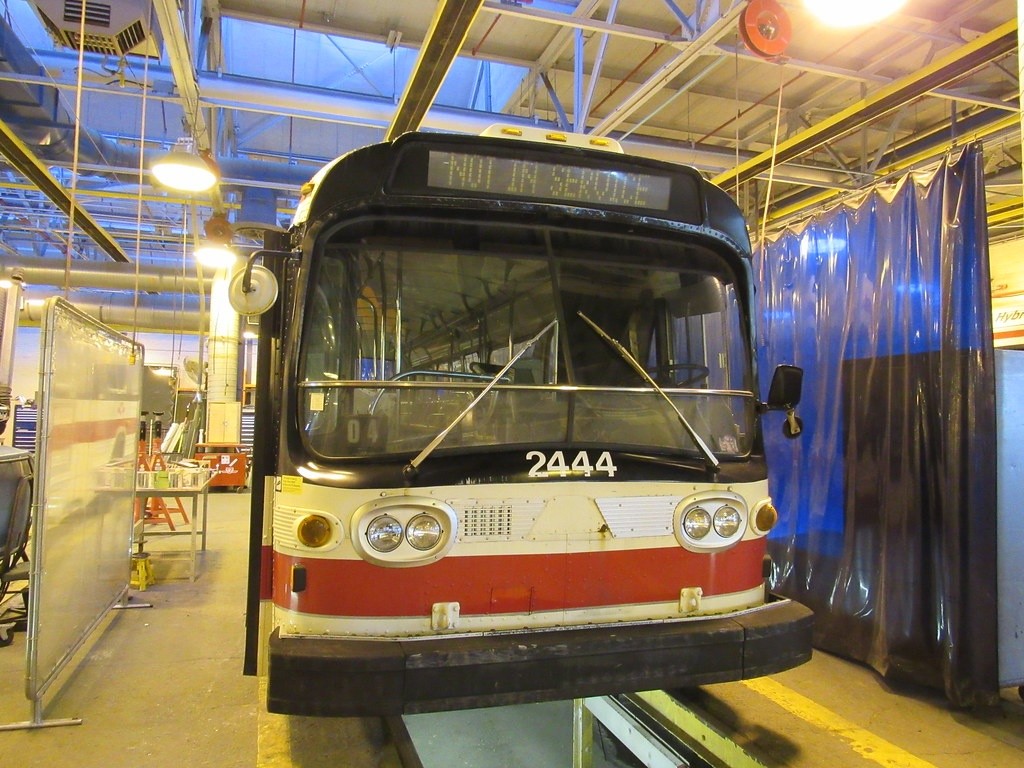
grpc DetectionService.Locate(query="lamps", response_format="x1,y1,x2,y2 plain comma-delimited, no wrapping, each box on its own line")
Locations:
151,85,216,192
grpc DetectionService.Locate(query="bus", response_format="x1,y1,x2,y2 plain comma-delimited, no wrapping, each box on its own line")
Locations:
224,119,819,726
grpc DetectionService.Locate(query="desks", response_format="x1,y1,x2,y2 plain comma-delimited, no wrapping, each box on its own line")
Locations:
134,471,219,582
97,487,136,571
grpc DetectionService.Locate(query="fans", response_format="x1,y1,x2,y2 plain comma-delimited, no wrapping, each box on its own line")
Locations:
183,357,209,385
